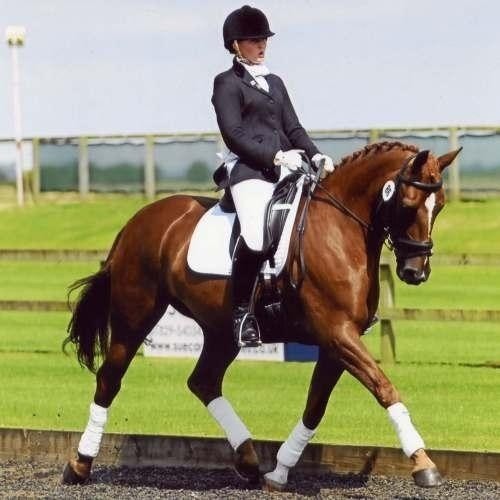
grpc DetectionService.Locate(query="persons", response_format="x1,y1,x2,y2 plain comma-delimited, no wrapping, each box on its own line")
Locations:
210,3,336,347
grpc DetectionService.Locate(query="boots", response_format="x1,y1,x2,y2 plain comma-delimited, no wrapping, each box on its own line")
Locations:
219,233,266,346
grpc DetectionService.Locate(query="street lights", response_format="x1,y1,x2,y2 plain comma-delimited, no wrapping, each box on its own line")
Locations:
7,25,26,202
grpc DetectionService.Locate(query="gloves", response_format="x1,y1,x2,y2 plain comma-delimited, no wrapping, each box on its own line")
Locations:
310,152,334,174
272,148,305,174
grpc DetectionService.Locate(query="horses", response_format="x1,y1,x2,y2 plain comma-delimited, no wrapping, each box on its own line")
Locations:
61,141,463,492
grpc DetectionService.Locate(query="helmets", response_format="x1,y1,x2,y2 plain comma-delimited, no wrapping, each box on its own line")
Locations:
222,5,275,53
